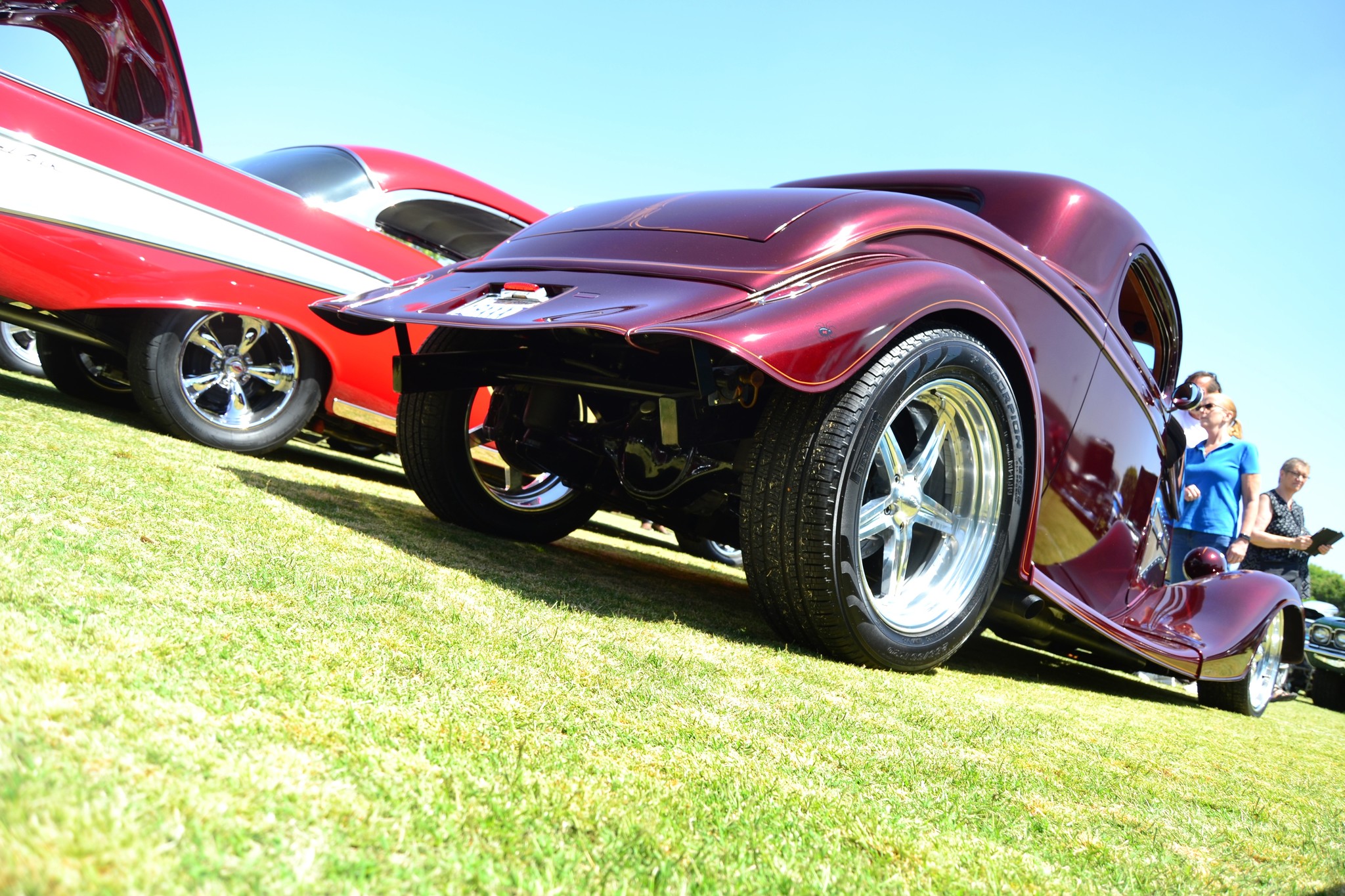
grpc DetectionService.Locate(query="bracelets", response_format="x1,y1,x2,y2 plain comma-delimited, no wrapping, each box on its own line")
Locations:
1238,533,1251,541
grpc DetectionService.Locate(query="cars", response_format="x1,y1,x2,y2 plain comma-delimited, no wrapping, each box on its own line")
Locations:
0,0,555,481
306,166,1307,719
1304,616,1345,713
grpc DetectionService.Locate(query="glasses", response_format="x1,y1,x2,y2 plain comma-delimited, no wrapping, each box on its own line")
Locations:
1287,469,1310,481
1195,403,1228,411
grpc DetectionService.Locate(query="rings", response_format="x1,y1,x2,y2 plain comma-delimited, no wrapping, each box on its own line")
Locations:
1188,494,1192,497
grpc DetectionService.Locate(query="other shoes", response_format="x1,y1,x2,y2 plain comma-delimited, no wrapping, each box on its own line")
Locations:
1270,687,1297,701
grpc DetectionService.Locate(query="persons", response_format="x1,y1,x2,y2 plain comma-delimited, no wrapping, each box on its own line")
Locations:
1236,457,1334,702
1170,394,1261,586
1160,370,1223,541
